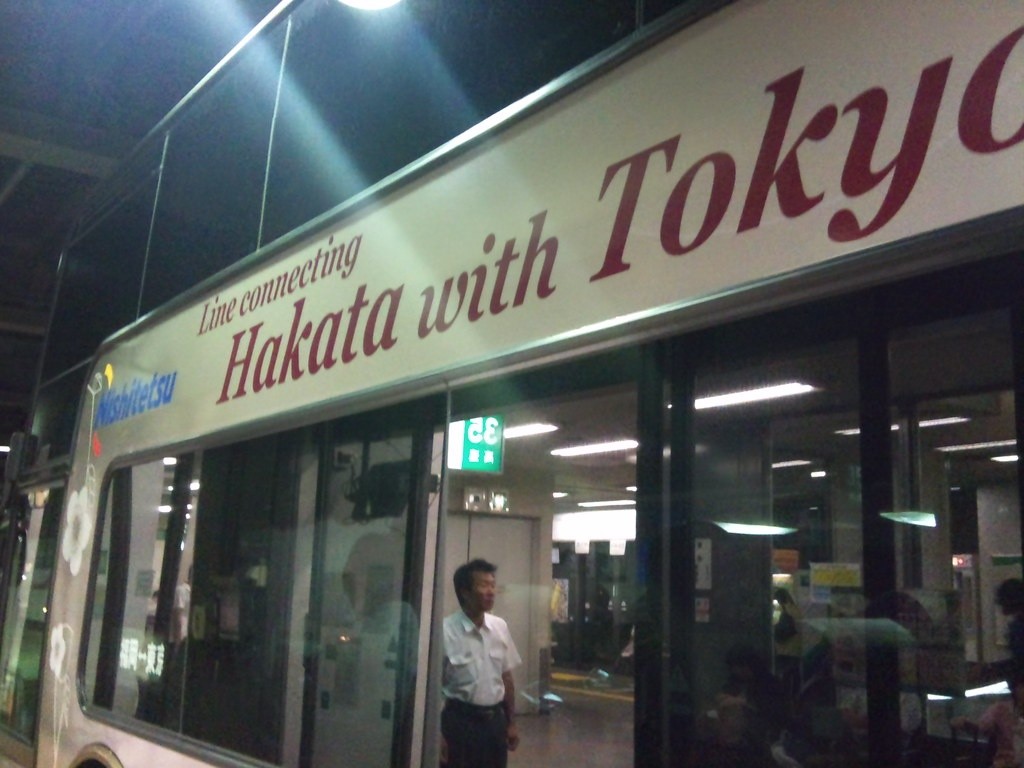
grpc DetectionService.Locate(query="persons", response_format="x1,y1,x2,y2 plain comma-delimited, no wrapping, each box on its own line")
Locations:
950,578,1024,768
147,564,194,684
319,533,418,706
443,559,523,768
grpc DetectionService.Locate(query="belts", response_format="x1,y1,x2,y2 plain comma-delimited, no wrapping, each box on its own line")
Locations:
471,707,498,720
